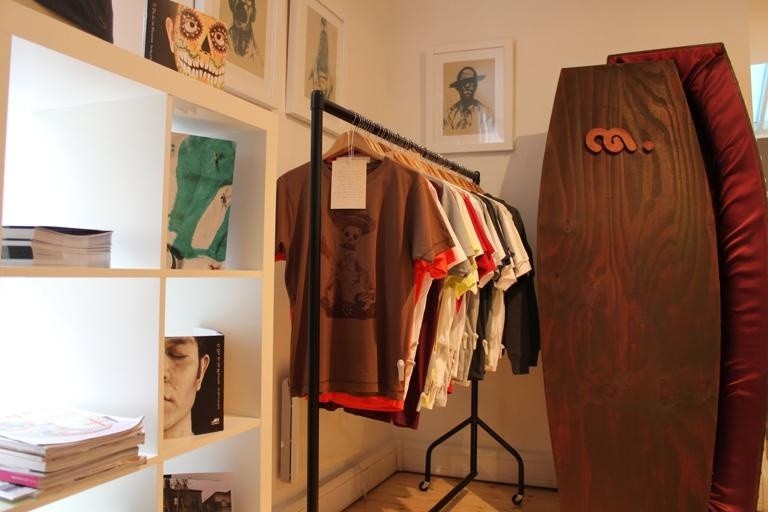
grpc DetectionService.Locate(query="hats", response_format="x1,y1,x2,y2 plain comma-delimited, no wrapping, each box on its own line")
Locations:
449,67,484,87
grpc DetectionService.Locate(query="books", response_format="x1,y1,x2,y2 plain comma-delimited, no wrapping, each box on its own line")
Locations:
2,224,113,267
0,411,148,502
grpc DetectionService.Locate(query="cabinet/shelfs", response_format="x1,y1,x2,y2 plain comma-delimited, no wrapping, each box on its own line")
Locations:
1,1,279,512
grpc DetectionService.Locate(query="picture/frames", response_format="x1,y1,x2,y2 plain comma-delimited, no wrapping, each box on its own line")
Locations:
286,1,349,139
192,0,281,113
424,39,514,151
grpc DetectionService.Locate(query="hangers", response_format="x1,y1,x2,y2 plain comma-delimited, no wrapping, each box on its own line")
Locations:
277,108,499,201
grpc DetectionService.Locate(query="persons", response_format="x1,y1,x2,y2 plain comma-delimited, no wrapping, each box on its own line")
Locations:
165,4,228,92
225,0,260,68
165,336,210,437
443,66,495,134
